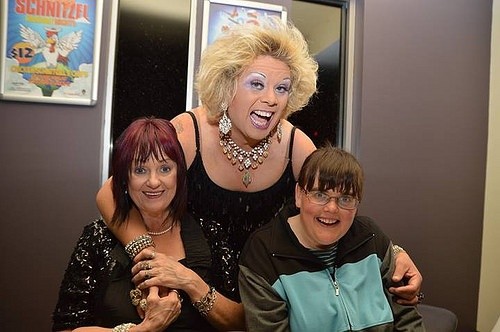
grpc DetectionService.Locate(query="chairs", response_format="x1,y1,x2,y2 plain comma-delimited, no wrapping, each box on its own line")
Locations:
417,305,459,332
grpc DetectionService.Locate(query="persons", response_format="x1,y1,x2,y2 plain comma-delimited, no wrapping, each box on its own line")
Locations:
96,15,422,319
53,117,223,332
238,138,429,332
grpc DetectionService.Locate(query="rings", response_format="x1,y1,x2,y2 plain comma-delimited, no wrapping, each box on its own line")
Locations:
143,270,150,278
418,293,425,301
149,250,156,258
170,290,179,295
180,298,183,302
177,294,180,298
139,299,146,309
143,260,152,270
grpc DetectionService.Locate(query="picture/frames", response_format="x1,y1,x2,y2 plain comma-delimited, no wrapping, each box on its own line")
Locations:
0,0,104,108
199,0,288,112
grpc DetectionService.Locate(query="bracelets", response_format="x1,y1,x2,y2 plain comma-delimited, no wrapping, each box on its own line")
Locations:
112,323,137,332
393,245,404,255
125,235,156,259
192,286,217,316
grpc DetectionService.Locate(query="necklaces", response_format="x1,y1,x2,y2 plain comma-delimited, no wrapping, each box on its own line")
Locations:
219,133,271,189
147,220,176,235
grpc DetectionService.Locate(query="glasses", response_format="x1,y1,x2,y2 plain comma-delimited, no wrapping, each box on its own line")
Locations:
300,185,359,210
